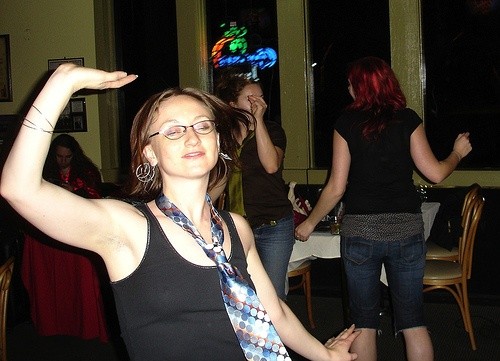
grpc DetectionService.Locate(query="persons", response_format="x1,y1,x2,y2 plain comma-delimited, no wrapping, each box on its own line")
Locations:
1,63,363,361
217,66,296,299
40,133,102,182
294,58,473,360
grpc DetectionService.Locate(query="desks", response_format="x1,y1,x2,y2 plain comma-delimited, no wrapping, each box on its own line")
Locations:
286,203,441,336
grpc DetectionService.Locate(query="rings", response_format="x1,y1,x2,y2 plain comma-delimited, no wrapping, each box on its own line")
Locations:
296,236,299,238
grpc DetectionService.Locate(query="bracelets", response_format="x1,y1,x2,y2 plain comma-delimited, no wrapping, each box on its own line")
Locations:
451,149,463,160
22,103,55,134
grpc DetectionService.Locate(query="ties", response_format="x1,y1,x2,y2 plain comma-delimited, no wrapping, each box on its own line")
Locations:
226,132,255,216
154,192,293,361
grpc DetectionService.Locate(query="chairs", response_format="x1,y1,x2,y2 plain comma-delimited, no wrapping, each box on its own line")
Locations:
287,260,315,329
423,195,486,351
0,255,15,361
425,183,480,312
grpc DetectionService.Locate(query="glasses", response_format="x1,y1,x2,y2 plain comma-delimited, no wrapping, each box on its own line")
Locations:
148,120,216,140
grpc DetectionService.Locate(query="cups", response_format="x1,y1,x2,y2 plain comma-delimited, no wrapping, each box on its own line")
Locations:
330,219,340,235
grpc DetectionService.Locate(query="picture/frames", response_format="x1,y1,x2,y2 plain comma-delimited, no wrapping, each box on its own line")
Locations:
48,57,84,80
52,98,87,133
0,34,13,102
